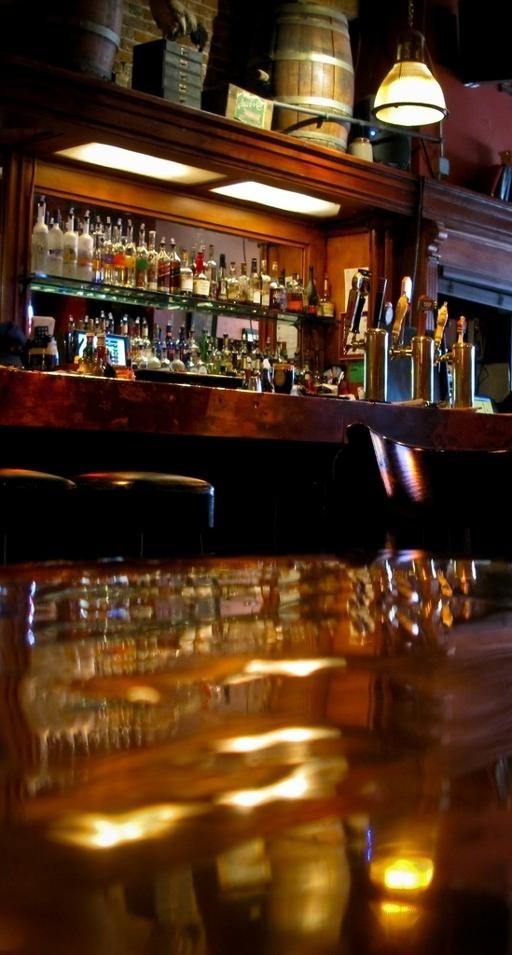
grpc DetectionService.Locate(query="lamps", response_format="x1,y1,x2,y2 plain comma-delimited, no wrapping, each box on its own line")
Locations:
368,0,451,128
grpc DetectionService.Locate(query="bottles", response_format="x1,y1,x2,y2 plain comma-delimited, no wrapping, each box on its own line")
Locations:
29,195,333,397
347,136,374,163
19,562,480,795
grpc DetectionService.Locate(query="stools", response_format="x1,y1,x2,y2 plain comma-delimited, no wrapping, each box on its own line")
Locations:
0,467,76,562
77,466,215,555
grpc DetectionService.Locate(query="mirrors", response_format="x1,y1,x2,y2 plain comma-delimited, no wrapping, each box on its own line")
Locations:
27,186,309,374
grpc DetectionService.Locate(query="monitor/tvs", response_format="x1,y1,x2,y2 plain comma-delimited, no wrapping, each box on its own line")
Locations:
72,329,132,371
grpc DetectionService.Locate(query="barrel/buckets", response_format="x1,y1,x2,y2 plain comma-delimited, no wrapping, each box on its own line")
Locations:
267,4,355,153
43,0,123,80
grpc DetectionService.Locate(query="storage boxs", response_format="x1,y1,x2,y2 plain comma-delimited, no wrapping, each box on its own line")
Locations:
202,82,275,131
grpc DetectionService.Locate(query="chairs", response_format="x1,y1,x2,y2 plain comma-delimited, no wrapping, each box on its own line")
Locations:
338,419,512,551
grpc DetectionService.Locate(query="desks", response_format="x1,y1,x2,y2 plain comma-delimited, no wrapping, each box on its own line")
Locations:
0,555,511,955
